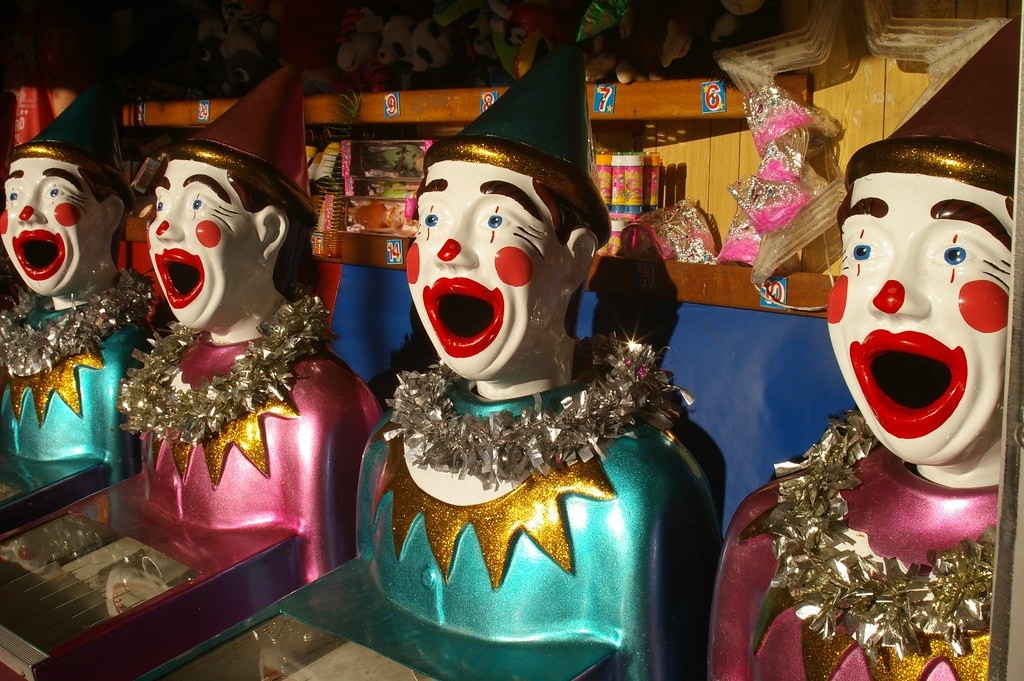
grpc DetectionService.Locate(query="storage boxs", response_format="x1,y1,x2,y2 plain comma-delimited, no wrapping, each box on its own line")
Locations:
339,137,439,269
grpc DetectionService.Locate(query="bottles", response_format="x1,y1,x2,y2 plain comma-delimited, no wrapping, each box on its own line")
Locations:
589,149,663,257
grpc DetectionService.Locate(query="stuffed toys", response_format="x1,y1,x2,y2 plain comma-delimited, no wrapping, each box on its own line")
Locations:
335,0,775,93
113,0,285,100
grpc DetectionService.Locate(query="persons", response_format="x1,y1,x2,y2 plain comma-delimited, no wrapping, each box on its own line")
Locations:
117,56,384,580
0,0,98,182
708,134,1016,681
0,80,152,479
355,48,721,681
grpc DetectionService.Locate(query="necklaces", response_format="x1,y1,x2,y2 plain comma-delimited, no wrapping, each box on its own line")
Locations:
0,270,155,376
117,295,328,441
775,410,987,652
387,331,673,476
